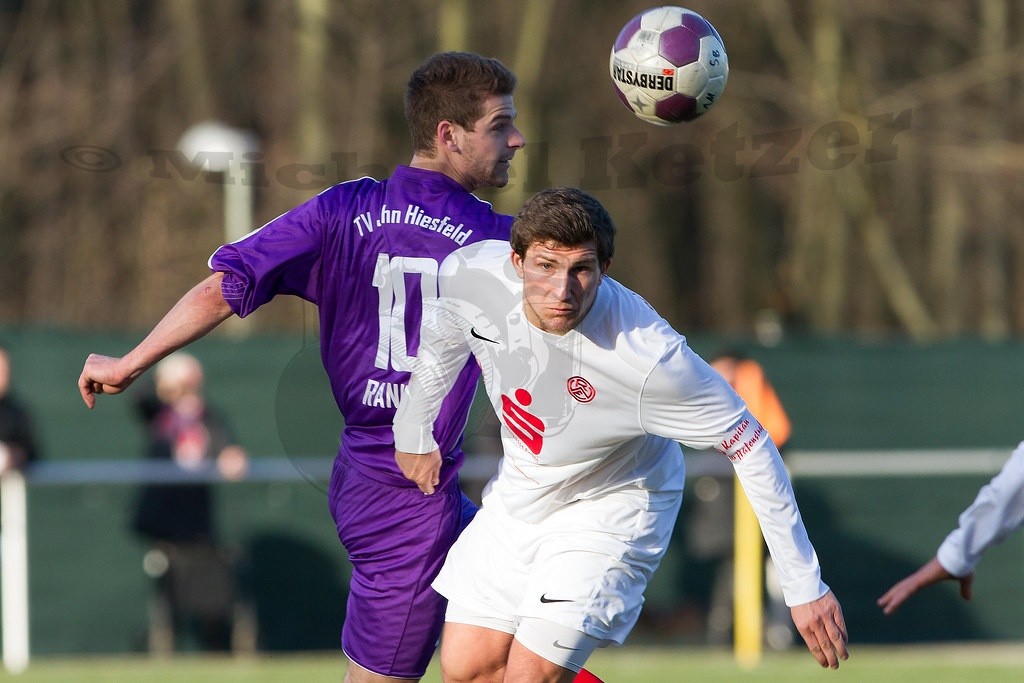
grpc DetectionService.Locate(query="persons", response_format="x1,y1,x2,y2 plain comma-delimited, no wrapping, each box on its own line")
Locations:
875,439,1024,614
126,351,248,653
76,53,526,683
392,187,848,683
0,349,45,481
679,351,794,641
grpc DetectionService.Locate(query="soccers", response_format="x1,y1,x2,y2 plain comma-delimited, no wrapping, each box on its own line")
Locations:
610,5,730,128
